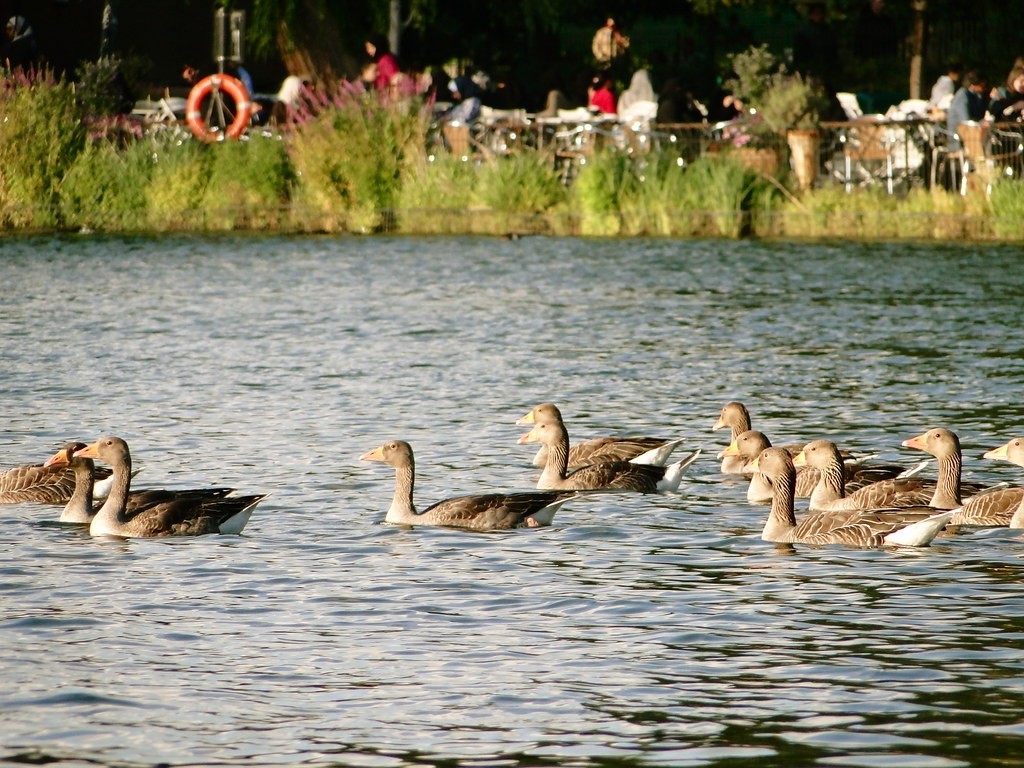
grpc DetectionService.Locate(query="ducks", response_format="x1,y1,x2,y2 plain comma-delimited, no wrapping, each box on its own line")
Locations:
0,401,1024,555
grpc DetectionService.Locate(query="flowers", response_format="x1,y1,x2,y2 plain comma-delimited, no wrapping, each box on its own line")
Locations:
719,96,777,150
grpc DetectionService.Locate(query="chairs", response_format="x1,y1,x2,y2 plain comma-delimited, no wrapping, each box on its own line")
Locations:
426,106,1024,195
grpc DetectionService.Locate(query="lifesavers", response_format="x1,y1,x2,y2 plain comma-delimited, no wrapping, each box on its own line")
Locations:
185,73,252,144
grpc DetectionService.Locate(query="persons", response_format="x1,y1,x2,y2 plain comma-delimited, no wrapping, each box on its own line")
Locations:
586,74,621,115
930,58,1024,156
590,15,629,71
363,33,504,111
269,75,319,129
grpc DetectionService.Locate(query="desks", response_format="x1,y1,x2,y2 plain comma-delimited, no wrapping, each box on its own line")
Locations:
654,120,717,160
818,117,936,195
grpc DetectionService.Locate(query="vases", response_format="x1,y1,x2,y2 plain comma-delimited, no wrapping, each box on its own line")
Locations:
728,145,783,184
